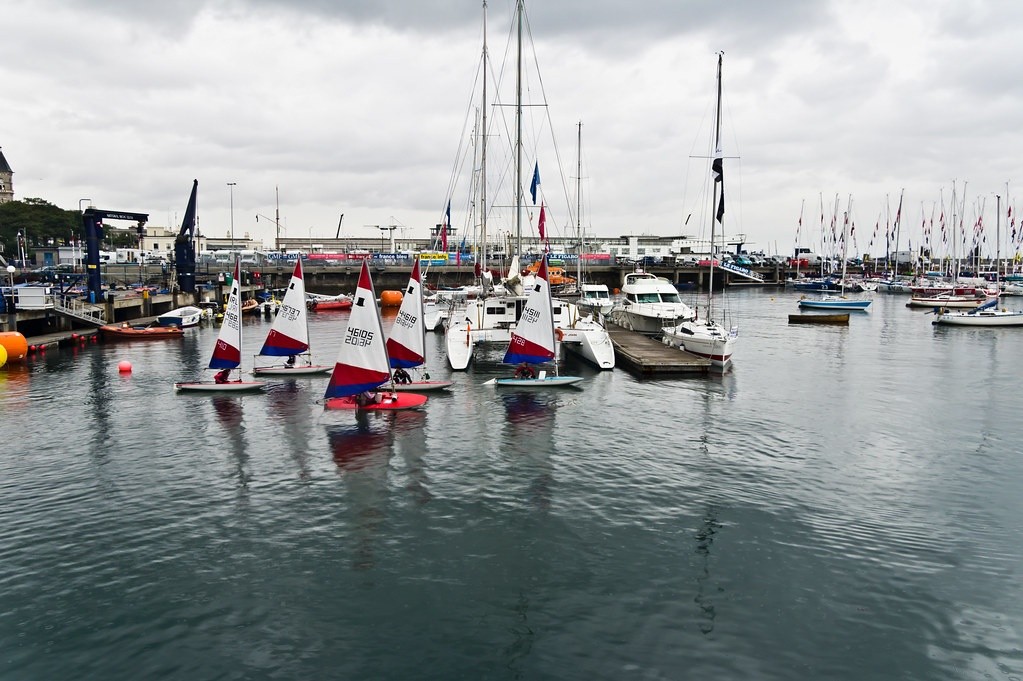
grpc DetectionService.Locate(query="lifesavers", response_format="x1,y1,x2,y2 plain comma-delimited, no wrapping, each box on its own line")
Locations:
554,329,563,341
562,278,567,284
246,300,252,305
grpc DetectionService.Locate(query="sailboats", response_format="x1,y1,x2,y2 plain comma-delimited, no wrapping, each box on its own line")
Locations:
173,255,267,391
780,179,1023,327
419,0,615,386
322,257,428,410
368,258,457,391
249,258,335,375
660,50,739,368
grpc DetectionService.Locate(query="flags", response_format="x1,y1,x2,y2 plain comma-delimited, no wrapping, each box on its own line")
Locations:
530,161,541,204
455,238,465,269
537,202,547,241
440,199,451,254
789,196,1022,277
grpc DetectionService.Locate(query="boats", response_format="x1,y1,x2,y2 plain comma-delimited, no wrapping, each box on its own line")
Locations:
605,267,696,337
305,292,354,310
99,292,282,339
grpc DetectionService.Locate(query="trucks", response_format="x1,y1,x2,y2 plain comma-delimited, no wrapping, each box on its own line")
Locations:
723,254,768,267
199,249,302,266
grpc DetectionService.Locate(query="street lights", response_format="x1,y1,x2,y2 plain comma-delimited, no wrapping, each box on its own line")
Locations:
226,182,236,251
17,227,29,261
7,265,16,303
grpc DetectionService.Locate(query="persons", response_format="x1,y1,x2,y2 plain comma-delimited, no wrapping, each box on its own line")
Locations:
285,354,296,369
514,361,537,380
359,390,376,406
393,368,413,385
213,369,232,384
